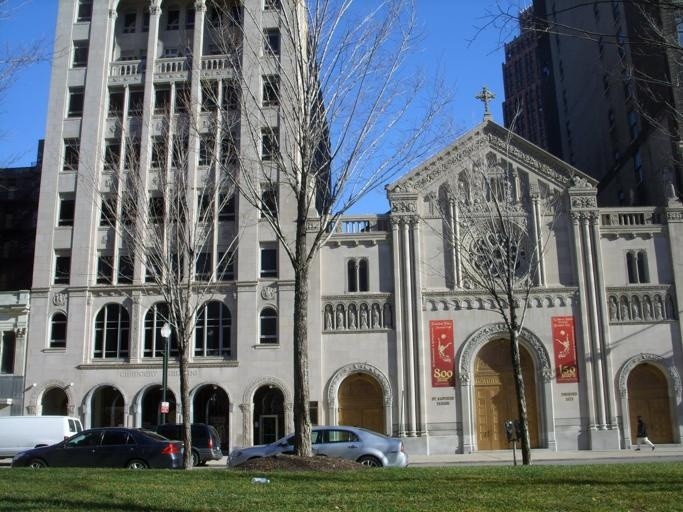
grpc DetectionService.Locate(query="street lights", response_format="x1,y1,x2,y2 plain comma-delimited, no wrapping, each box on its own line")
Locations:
160,323,172,425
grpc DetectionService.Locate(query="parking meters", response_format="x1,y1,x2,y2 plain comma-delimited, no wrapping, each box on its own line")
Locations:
504,420,521,466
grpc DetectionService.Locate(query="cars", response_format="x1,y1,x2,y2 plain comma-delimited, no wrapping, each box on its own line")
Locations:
227,426,408,466
0,415,223,469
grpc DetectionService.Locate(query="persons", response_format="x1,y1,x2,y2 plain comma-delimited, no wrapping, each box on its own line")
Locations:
634,415,656,452
